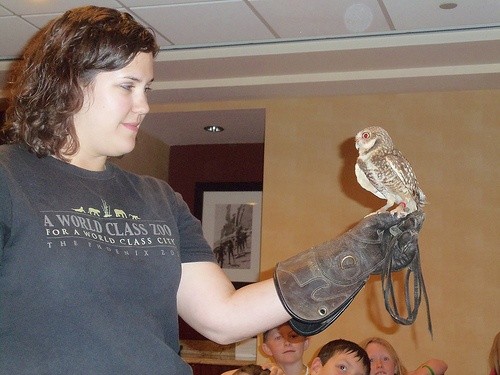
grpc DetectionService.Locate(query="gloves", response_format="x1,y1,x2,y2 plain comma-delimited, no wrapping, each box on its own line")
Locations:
271,206,433,341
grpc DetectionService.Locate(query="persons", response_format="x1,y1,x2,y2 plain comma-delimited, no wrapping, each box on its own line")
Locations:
0,6,433,375
221,321,448,375
214,214,247,268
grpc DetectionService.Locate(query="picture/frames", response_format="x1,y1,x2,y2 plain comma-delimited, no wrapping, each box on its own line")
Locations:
194,182,263,291
